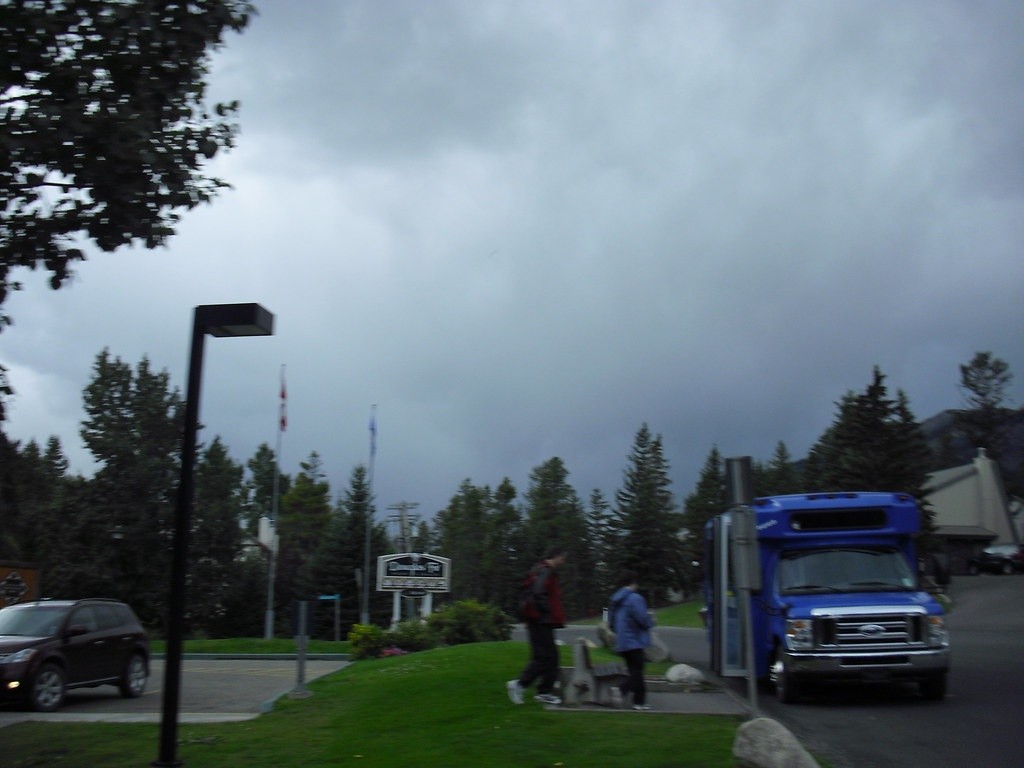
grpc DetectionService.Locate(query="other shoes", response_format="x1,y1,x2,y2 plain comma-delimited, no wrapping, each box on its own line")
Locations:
633,703,654,710
610,687,623,708
535,693,563,704
506,679,525,704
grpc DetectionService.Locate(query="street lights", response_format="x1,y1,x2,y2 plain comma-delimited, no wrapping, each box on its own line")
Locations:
152,303,276,768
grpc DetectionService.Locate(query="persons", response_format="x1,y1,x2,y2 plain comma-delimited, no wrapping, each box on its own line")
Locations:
505,543,568,706
606,570,659,711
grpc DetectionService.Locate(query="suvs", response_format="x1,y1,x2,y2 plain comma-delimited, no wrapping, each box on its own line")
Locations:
0,598,150,715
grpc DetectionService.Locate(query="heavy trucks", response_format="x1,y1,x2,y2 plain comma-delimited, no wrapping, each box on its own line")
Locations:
699,492,956,707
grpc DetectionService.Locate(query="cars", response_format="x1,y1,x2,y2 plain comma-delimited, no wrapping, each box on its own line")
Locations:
980,543,1024,575
